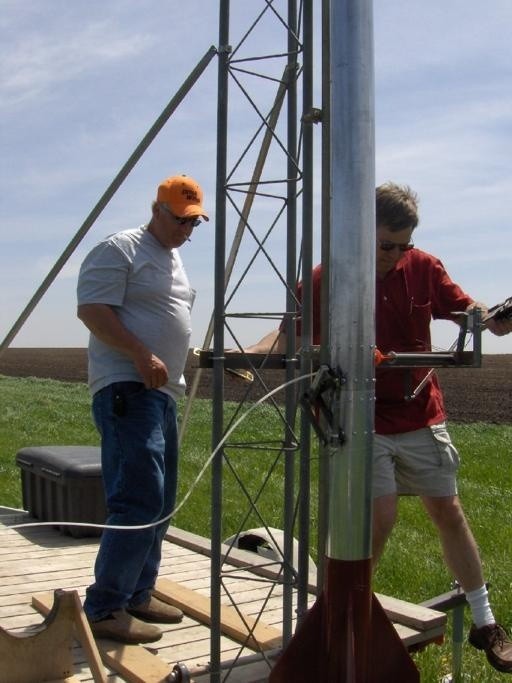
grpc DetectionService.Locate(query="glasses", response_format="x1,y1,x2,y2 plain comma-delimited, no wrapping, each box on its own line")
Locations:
160,204,201,226
377,238,415,252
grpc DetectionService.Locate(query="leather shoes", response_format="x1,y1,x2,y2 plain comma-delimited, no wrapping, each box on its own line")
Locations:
129,595,184,624
468,623,512,674
81,607,163,643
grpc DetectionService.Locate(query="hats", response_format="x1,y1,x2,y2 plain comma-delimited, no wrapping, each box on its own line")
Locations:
157,173,210,221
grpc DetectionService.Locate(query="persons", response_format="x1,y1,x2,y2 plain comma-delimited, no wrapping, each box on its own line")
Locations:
225,180,512,673
77,174,212,642
